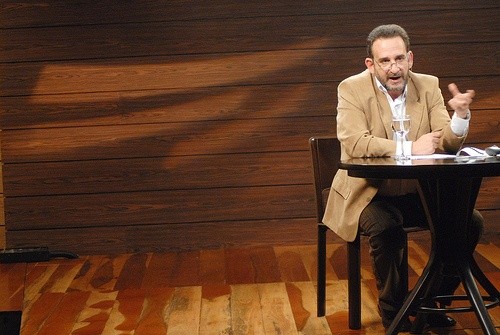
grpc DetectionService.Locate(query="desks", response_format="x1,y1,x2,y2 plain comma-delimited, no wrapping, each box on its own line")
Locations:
338,153,500,334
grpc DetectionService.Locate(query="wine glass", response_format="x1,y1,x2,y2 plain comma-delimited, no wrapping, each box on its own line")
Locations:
391,115,411,160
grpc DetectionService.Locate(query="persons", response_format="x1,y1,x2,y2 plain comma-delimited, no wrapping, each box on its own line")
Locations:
322,24,485,331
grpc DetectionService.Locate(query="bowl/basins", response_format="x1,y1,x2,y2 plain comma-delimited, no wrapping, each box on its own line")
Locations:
485,147,500,156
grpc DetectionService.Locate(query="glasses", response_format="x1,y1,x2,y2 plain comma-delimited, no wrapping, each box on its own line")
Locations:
374,52,409,71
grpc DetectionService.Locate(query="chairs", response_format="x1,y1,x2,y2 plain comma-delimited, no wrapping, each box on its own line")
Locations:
311,133,433,330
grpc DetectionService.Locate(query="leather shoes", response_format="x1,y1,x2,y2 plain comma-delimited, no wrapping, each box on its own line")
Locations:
413,301,458,327
376,303,411,332
